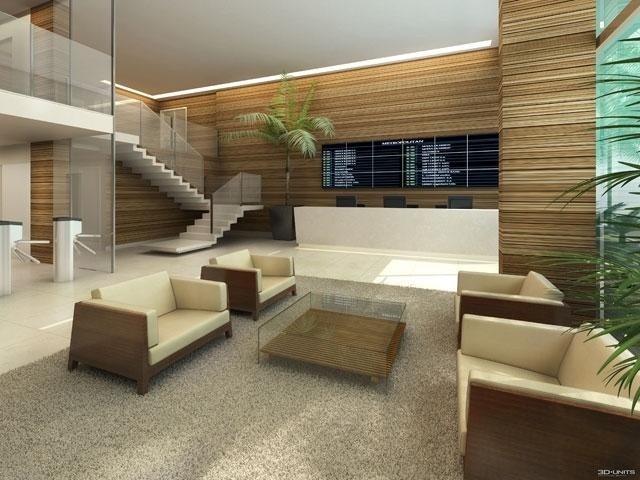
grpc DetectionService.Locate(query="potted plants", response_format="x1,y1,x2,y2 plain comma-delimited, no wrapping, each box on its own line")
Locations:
213,68,339,242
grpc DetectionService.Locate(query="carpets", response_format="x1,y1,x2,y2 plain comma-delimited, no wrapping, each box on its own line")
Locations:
1,275,461,480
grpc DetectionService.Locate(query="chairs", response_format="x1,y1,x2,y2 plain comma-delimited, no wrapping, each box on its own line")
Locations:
334,191,475,211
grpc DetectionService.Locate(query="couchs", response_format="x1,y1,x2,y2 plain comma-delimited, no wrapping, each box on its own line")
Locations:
198,246,298,322
65,269,234,398
449,311,640,480
451,267,570,353
